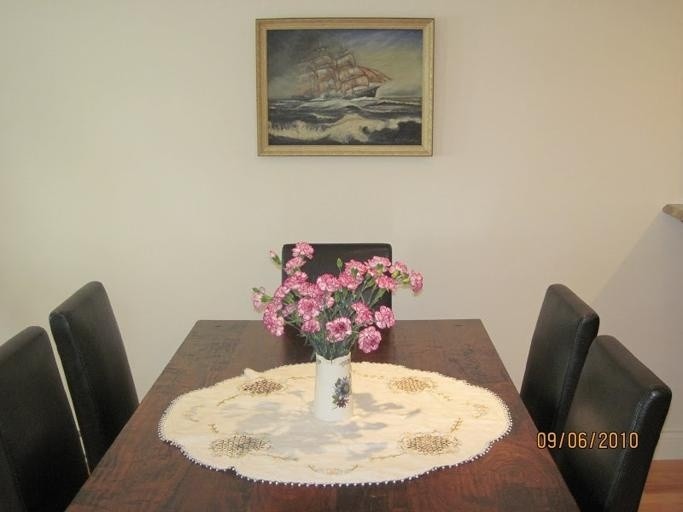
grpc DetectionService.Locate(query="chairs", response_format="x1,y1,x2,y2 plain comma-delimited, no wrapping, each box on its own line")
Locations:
521,284,671,512
278,246,392,323
1,282,140,512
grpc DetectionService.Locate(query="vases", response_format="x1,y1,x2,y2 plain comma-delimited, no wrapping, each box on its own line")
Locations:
313,353,355,422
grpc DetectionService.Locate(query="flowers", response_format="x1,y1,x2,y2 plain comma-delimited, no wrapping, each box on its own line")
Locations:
250,244,425,361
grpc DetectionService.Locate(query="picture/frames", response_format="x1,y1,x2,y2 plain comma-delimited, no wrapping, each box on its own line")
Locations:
256,19,435,158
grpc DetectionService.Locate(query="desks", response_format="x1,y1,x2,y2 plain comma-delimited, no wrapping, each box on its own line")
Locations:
65,320,583,509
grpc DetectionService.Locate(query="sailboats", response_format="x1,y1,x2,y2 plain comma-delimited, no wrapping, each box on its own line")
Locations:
295,44,394,102
